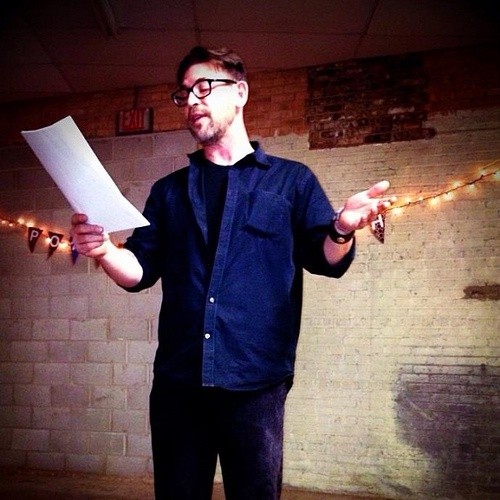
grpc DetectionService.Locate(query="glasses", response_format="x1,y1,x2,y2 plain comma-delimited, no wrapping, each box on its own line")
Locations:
172,78,237,107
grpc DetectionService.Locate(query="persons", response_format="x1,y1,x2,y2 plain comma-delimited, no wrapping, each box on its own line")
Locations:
70,43,397,500
